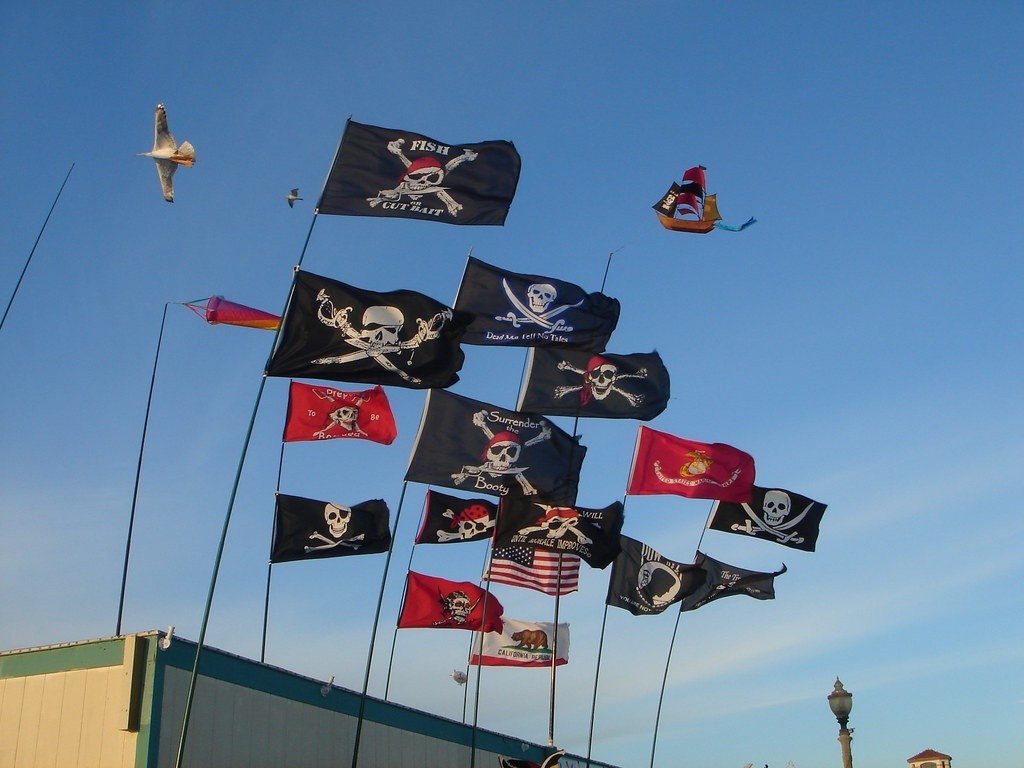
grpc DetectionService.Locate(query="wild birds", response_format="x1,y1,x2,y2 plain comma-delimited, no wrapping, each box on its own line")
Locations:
282,185,303,212
135,102,196,203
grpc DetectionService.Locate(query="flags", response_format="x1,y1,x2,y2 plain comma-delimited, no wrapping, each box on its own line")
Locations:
604,535,705,618
266,269,479,391
452,257,622,353
269,492,392,564
519,349,672,421
399,573,506,633
402,388,586,506
707,485,829,552
412,489,498,542
680,552,788,611
469,618,572,668
283,382,399,446
316,119,523,226
626,425,757,503
491,496,624,566
483,541,581,596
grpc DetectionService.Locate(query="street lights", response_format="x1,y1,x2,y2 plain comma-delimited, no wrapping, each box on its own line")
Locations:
827,675,854,768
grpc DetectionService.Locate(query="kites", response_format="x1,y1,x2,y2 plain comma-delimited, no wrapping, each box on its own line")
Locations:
651,167,757,234
